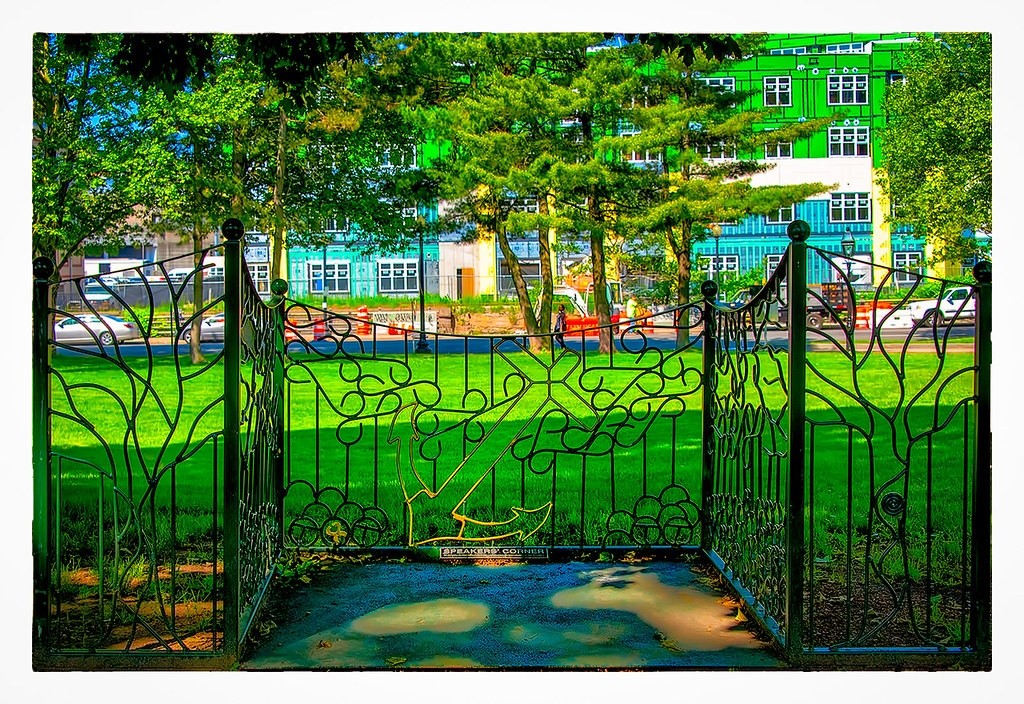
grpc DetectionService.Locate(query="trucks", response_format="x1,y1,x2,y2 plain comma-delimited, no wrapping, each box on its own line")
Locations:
728,282,850,331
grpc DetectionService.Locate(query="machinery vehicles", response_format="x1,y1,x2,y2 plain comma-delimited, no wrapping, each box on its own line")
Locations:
534,280,625,335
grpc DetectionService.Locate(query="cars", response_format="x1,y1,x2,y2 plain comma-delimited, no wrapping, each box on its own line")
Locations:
177,312,224,344
167,268,194,281
54,314,139,346
83,282,120,307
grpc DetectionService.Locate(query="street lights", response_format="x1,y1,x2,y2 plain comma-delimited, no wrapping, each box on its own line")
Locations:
842,226,855,353
712,223,722,301
417,215,432,354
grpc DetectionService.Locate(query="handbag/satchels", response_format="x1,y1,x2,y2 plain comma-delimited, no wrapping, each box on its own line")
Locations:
561,324,566,333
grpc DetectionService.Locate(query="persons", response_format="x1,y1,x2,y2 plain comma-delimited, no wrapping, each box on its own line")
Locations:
554,304,567,350
627,294,638,333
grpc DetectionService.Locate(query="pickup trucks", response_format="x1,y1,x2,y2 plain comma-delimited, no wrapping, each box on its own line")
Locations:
906,287,976,329
